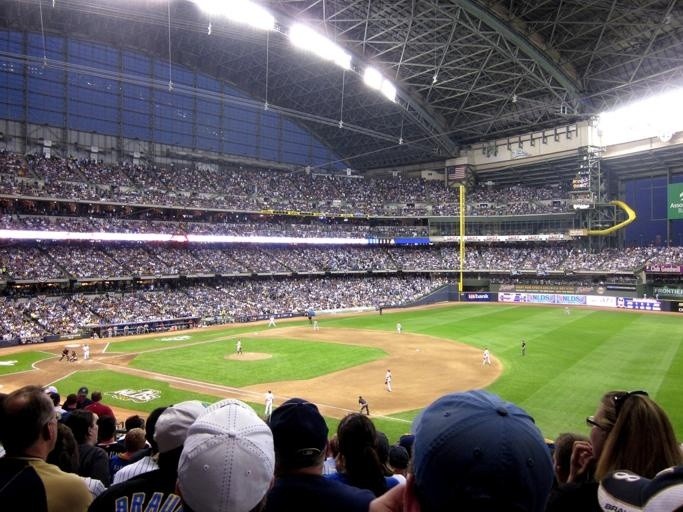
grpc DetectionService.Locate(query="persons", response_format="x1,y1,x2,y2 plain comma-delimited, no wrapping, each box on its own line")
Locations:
383,369,393,392
67,350,78,362
81,344,90,360
520,339,526,356
234,338,243,358
588,243,682,275
59,347,69,361
479,346,491,369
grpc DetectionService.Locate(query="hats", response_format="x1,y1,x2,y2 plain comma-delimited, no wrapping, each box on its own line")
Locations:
78,387,88,396
83,403,116,428
176,398,276,512
145,404,173,449
267,397,330,466
596,462,683,512
388,434,416,469
409,389,556,512
43,386,59,394
153,400,214,453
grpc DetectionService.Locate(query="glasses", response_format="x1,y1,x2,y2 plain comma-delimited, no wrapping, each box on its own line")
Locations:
585,416,608,430
613,389,650,419
